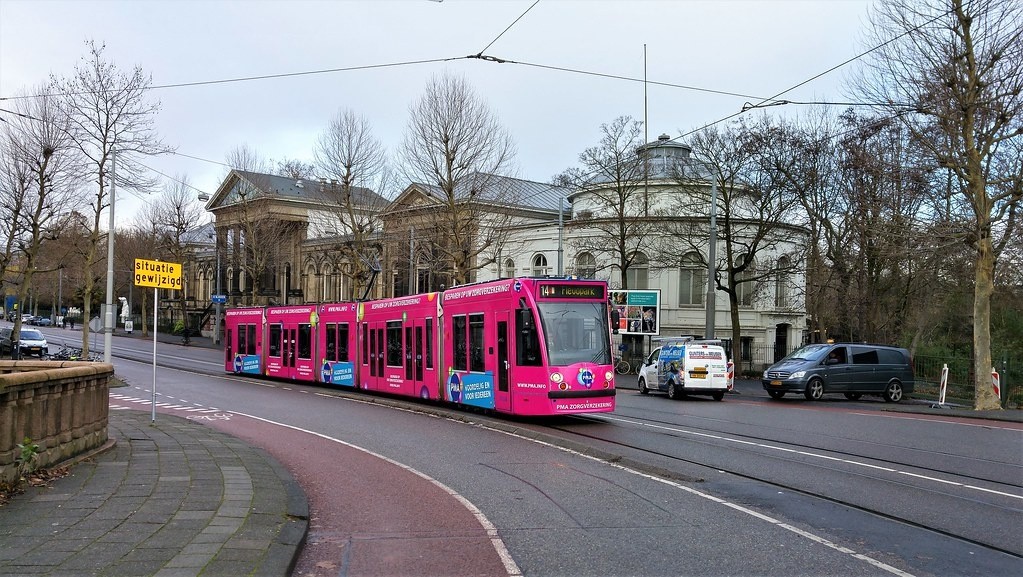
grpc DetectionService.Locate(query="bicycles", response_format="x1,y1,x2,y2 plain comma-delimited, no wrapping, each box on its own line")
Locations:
44,345,101,363
612,353,630,375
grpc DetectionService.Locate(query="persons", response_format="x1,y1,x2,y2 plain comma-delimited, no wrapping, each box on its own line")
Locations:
61,315,68,330
69,316,75,329
822,349,844,364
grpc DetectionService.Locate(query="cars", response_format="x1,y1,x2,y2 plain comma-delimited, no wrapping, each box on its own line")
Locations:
0,308,52,326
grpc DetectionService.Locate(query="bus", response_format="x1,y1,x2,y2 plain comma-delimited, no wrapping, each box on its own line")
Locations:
222,274,614,419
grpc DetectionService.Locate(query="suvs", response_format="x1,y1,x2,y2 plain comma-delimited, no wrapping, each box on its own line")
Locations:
637,336,728,400
763,341,915,402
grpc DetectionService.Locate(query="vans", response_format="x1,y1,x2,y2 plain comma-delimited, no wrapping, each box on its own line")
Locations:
0,326,47,355
